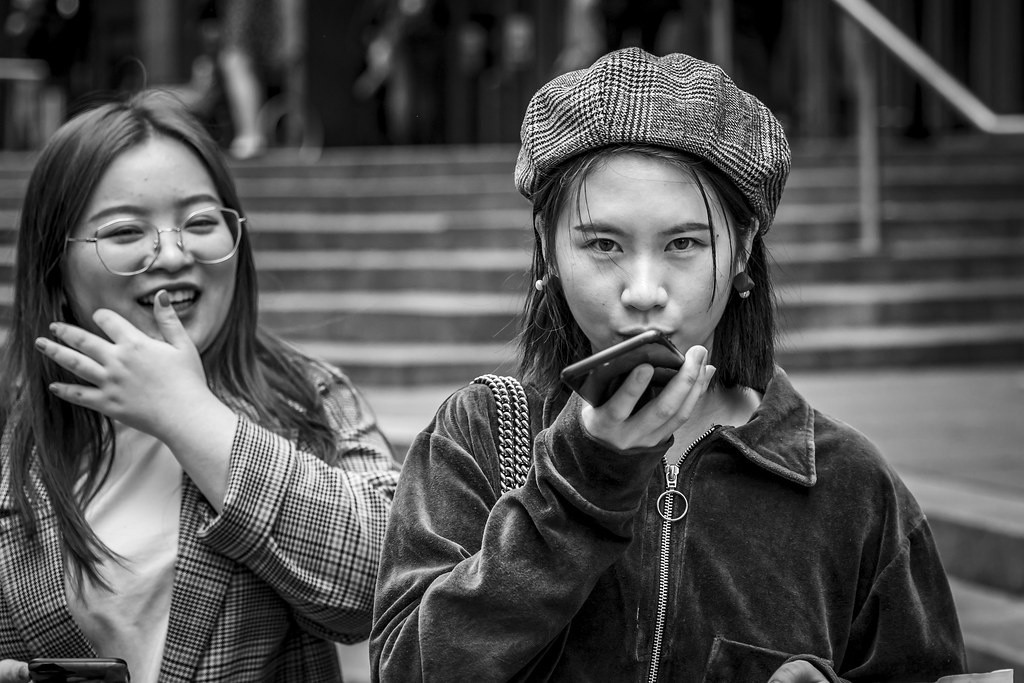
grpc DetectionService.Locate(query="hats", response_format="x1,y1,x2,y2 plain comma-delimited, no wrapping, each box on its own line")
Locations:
514,47,793,236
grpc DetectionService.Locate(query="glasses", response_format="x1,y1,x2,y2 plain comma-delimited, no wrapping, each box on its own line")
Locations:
69,206,248,276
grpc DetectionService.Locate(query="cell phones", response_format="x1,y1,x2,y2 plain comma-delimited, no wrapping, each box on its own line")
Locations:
29,658,131,683
560,329,686,416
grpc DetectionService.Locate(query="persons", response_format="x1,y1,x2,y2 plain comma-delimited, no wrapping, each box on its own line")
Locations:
0,89,407,683
368,48,969,683
